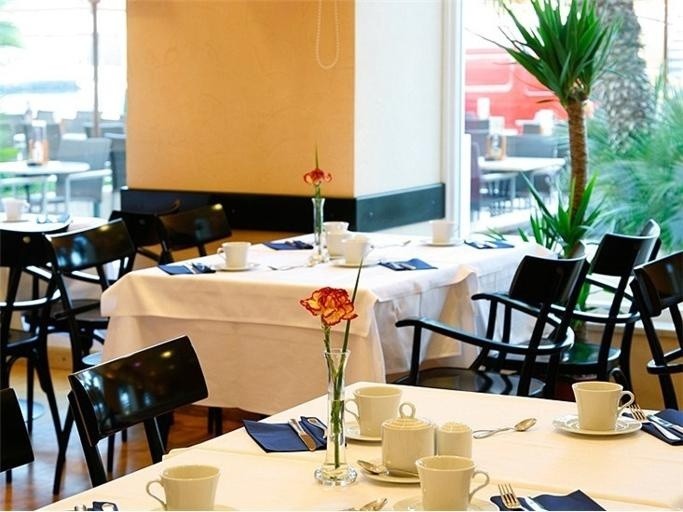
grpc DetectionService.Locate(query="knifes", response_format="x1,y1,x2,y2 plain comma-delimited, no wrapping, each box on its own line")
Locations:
524,494,549,511
647,412,682,431
286,418,316,451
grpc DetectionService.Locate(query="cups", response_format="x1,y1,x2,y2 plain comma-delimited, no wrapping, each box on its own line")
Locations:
144,463,219,510
570,380,634,431
413,455,489,510
429,219,458,244
1,197,30,220
380,402,434,475
322,218,371,264
345,387,402,436
216,241,251,267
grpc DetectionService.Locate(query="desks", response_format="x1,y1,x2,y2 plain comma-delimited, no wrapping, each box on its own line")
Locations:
35,380,682,512
123,230,555,416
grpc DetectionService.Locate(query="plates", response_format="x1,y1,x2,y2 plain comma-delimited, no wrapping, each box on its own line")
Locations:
152,503,234,509
393,495,500,511
208,264,258,272
345,420,383,443
419,239,461,247
1,218,29,223
551,413,642,438
332,260,380,267
359,462,420,483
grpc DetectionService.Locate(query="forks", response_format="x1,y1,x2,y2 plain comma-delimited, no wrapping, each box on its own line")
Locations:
497,483,526,511
474,417,537,437
628,403,678,442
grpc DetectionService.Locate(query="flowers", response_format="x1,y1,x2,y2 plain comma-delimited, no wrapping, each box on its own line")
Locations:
300,138,334,198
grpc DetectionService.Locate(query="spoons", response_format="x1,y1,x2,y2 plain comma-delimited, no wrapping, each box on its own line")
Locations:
340,496,387,510
358,457,417,477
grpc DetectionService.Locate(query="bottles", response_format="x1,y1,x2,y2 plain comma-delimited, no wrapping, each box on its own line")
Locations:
435,421,472,460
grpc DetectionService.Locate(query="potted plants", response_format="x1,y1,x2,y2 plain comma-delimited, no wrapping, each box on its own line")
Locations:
460,1,612,404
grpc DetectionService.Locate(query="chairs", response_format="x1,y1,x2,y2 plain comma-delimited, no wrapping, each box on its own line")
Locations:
1,218,70,485
630,250,683,412
483,218,661,408
67,333,210,487
391,253,591,398
1,209,109,326
